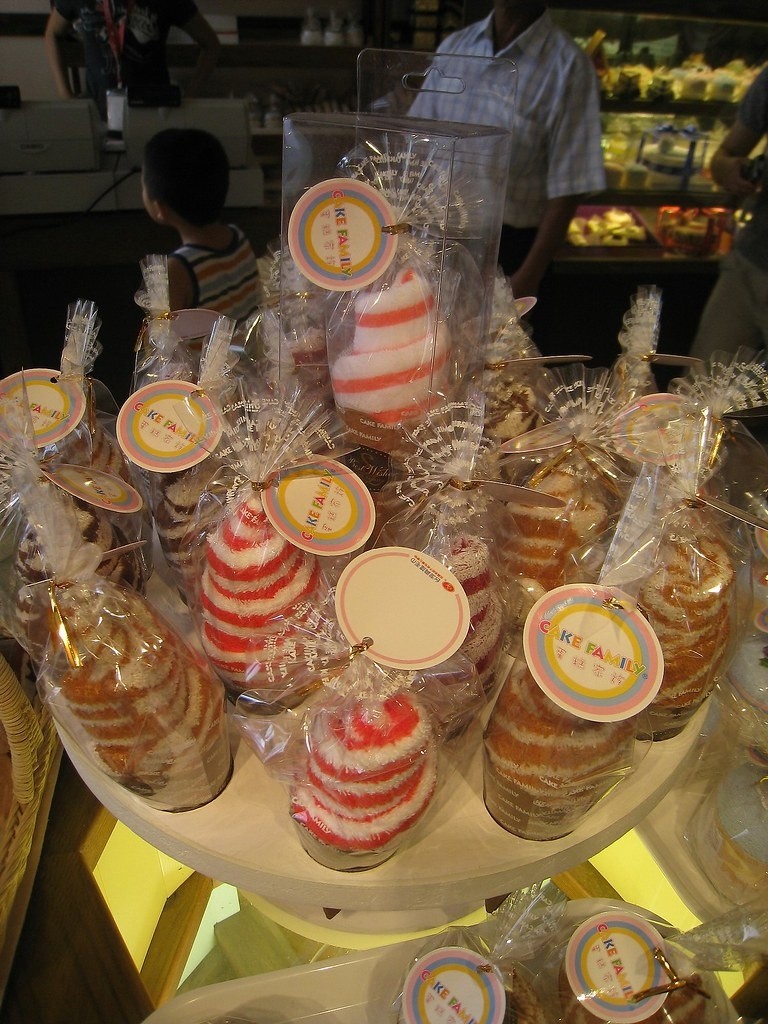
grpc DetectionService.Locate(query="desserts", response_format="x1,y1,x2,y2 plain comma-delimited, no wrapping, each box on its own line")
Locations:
0,234,768,1023
559,60,748,245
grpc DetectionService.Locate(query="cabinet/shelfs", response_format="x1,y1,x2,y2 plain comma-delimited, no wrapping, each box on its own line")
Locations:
546,9,767,264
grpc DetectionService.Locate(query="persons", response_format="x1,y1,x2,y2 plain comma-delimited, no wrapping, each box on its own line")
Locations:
44,0,223,101
406,0,605,299
140,127,262,360
689,67,768,379
